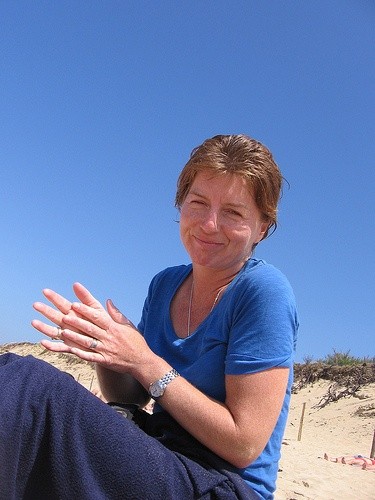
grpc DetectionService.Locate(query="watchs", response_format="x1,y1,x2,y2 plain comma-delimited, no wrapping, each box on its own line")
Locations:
150,369,179,399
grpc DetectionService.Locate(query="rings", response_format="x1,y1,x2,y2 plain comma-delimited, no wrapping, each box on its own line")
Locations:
89,340,99,348
53,328,63,341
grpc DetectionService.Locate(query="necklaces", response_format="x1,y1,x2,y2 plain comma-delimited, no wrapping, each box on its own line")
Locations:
186,279,223,336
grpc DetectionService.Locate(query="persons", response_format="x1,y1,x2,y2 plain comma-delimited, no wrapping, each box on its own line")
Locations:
0,134,301,500
